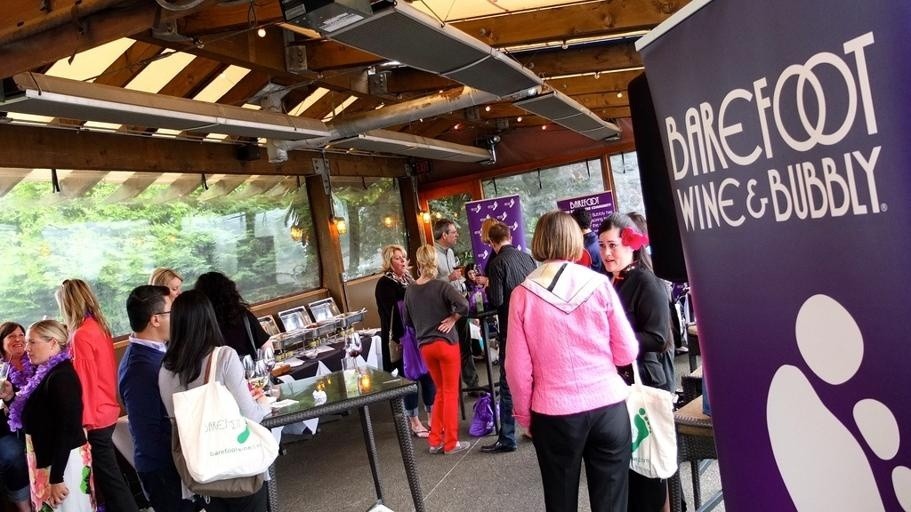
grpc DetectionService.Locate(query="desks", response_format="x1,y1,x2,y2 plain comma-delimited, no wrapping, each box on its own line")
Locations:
207,296,373,411
259,362,424,511
668,282,727,512
457,302,503,435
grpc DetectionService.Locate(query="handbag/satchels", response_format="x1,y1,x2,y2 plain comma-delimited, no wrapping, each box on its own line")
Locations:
388,305,404,364
166,346,279,499
397,301,429,381
624,359,680,481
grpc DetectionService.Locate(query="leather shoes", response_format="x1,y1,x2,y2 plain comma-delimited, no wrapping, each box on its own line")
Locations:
481,440,518,453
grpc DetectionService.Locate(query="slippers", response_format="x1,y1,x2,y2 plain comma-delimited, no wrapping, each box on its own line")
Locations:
410,423,432,438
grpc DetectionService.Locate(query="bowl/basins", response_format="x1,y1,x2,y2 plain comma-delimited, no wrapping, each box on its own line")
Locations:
305,350,319,359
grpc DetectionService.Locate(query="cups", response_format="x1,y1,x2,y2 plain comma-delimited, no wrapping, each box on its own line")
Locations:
474,291,484,312
341,358,359,396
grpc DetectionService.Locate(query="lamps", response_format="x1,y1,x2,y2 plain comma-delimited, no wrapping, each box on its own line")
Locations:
419,210,437,222
331,213,350,235
290,224,303,240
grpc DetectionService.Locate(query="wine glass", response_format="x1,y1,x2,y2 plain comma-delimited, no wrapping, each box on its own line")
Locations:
343,332,365,377
452,258,466,291
245,348,278,400
0,360,10,410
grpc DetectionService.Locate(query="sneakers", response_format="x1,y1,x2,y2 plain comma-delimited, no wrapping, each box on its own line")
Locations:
467,391,487,397
675,350,689,357
429,440,471,455
